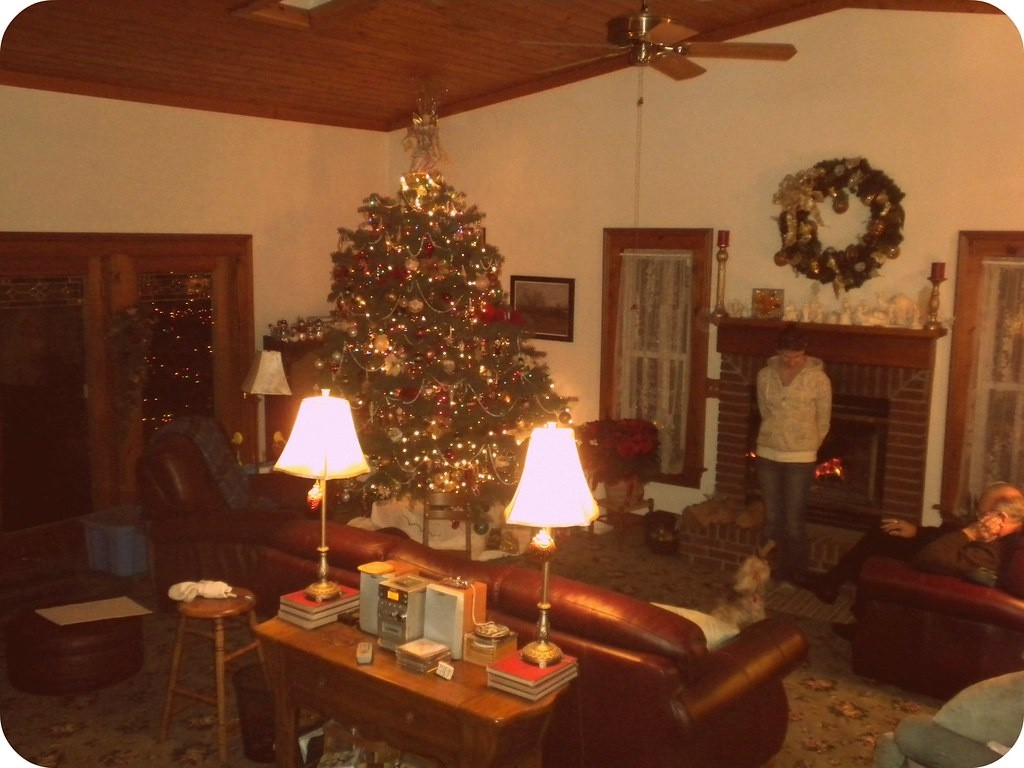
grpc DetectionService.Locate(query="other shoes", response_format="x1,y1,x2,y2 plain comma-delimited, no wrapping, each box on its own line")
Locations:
833,621,859,642
794,567,839,604
778,581,796,598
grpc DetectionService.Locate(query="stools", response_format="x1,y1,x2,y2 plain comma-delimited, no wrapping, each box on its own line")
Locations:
586,499,656,551
160,582,272,763
8,587,143,695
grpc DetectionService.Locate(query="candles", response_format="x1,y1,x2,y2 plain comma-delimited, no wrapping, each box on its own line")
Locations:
718,230,730,245
931,262,945,279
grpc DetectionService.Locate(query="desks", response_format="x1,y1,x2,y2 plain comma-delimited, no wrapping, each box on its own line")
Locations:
253,614,575,768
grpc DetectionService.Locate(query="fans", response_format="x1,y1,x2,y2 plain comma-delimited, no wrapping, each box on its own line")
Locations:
526,1,796,83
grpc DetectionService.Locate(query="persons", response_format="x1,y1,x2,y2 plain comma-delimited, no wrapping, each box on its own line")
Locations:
754,326,832,587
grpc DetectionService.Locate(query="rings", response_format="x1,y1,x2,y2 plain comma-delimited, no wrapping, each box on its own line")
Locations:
894,520,897,524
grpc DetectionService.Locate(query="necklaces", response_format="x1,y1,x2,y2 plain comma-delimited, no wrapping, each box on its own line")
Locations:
797,484,1024,644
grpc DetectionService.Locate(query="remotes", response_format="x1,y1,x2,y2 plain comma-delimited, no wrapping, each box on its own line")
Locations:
357,642,372,664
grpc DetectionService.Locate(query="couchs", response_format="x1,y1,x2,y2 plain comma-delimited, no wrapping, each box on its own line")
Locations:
262,508,814,768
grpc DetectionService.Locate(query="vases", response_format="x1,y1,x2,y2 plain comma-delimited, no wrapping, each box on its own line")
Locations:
602,472,641,511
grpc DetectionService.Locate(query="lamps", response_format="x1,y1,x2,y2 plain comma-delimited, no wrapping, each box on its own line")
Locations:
242,348,292,473
505,422,600,663
275,391,372,600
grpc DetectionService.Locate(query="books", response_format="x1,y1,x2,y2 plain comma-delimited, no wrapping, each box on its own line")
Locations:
278,583,360,630
484,648,579,702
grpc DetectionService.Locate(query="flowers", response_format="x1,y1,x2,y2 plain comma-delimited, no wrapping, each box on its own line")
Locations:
580,417,659,487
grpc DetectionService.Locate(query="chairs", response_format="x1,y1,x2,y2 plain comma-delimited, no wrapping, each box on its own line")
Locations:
141,414,329,579
869,668,1024,768
852,523,1023,688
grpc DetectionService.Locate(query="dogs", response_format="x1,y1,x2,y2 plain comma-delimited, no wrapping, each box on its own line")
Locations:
709,529,784,628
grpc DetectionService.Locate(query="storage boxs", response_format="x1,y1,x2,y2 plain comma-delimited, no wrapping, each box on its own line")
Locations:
81,502,163,577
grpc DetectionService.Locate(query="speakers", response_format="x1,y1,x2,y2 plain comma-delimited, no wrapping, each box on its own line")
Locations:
422,583,488,661
359,560,420,636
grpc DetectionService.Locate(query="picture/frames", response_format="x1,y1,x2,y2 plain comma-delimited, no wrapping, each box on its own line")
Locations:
508,274,575,341
